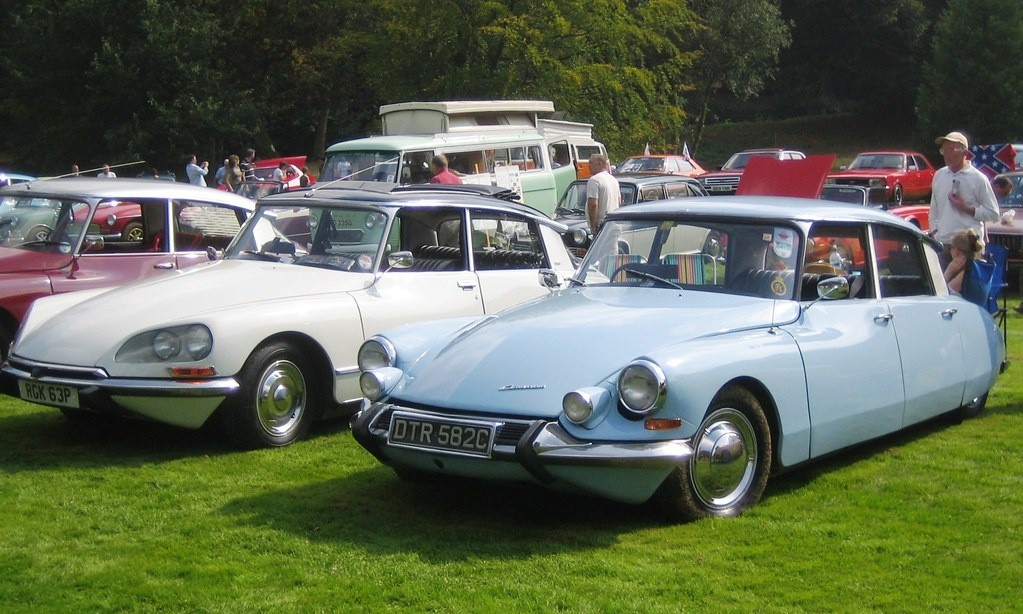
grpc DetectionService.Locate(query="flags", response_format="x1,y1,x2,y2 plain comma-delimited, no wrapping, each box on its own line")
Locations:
682,143,691,161
643,143,650,157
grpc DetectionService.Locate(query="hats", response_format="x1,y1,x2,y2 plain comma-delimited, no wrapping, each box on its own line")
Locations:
935,132,968,152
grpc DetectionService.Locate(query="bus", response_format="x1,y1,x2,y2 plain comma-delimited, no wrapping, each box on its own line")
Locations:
309,125,580,263
491,141,612,181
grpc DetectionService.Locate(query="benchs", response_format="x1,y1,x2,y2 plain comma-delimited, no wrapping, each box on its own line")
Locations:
147,230,233,253
849,274,924,298
408,244,542,273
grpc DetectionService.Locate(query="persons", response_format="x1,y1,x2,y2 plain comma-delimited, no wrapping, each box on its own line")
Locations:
943,227,986,298
136,148,354,205
65,164,84,178
430,154,463,185
991,174,1014,200
584,153,621,239
928,131,1001,259
98,164,118,177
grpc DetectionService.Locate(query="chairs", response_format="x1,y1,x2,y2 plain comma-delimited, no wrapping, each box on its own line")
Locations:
984,243,1010,370
959,257,996,308
742,269,818,302
816,273,883,300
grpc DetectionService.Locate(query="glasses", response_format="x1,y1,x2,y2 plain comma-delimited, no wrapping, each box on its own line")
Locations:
940,147,963,154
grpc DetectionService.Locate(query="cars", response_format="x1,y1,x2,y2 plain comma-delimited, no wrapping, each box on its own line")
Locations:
0,156,316,248
347,194,1006,525
804,179,934,277
0,176,275,367
981,171,1023,290
822,150,937,209
0,179,576,449
612,154,710,178
698,148,807,196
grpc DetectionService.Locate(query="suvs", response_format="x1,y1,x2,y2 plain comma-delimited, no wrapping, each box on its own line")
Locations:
548,173,711,261
